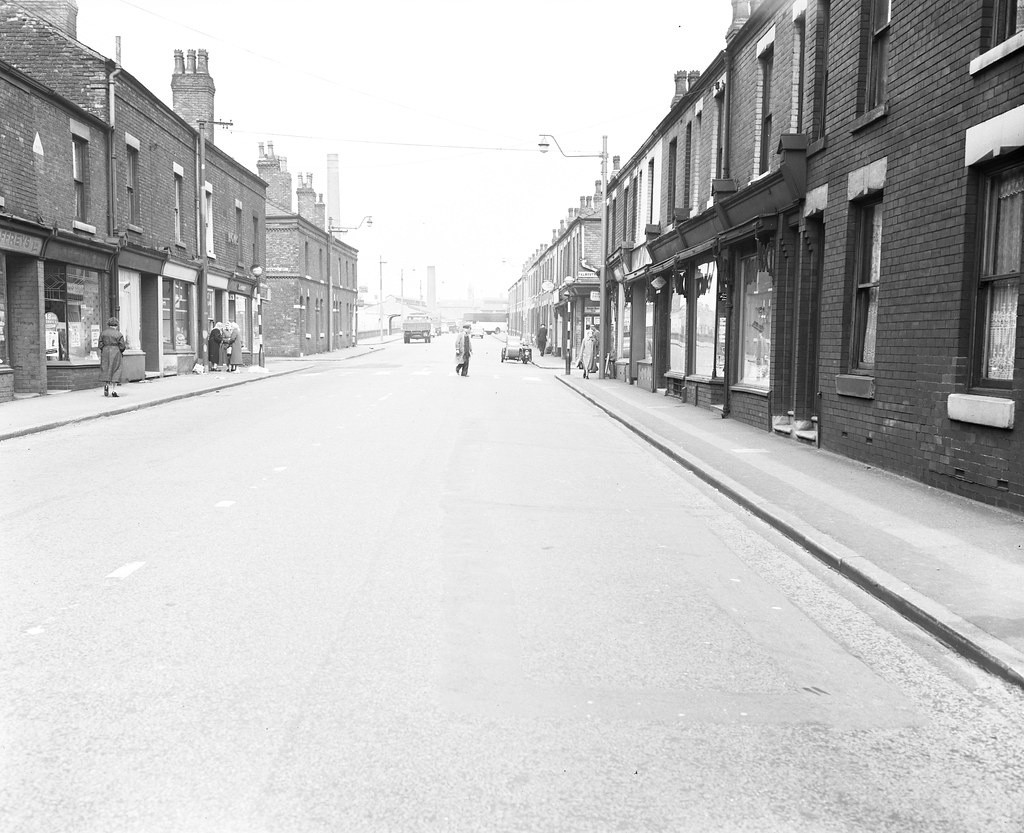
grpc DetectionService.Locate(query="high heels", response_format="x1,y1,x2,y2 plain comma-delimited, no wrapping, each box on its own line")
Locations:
104,385,108,395
112,390,120,396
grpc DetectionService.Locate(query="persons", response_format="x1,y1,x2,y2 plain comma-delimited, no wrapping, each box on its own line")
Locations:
580,323,600,379
97,316,126,397
537,323,547,357
455,324,474,377
207,321,242,373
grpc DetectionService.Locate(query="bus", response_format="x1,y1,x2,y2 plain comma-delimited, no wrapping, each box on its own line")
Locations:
463,311,507,335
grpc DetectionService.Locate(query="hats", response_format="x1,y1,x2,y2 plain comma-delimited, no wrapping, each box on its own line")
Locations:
463,323,472,329
540,323,546,325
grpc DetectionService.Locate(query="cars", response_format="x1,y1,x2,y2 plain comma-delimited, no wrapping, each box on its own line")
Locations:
471,324,484,340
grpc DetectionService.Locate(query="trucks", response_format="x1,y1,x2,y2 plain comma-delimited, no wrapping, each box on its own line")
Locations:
403,313,462,343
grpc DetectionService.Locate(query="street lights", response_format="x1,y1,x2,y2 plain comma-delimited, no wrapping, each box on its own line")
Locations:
564,275,575,376
252,265,265,368
538,133,608,381
328,214,373,357
399,268,416,332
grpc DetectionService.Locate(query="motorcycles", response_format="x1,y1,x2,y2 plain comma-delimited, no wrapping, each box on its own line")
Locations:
500,342,533,364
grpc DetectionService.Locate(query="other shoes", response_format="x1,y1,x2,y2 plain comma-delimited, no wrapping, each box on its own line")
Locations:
455,366,460,376
231,369,236,371
586,375,589,379
214,367,222,371
588,370,596,373
540,353,544,357
461,373,470,377
211,367,214,371
596,367,598,371
583,370,586,378
233,371,240,374
226,369,232,372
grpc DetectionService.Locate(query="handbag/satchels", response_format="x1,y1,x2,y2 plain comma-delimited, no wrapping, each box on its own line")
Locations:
226,346,232,355
577,360,583,369
